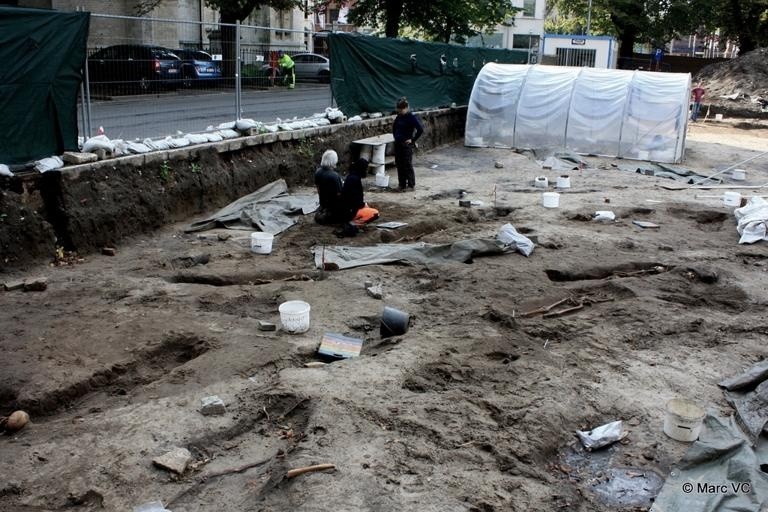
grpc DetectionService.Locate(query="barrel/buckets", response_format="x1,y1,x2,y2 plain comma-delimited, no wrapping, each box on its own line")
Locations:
375,174,389,187
664,397,704,443
724,192,743,207
279,300,310,334
544,192,560,208
381,306,409,336
251,232,273,255
733,168,745,181
715,114,723,121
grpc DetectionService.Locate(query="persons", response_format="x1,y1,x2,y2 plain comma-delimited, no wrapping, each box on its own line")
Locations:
691,81,704,121
312,149,343,223
274,48,296,89
391,95,422,191
341,157,380,225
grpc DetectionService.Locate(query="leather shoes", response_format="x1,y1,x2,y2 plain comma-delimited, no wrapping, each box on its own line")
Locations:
392,186,406,191
402,186,415,192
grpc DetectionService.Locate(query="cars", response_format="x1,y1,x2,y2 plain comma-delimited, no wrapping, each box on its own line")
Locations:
260,53,330,84
88,44,223,94
